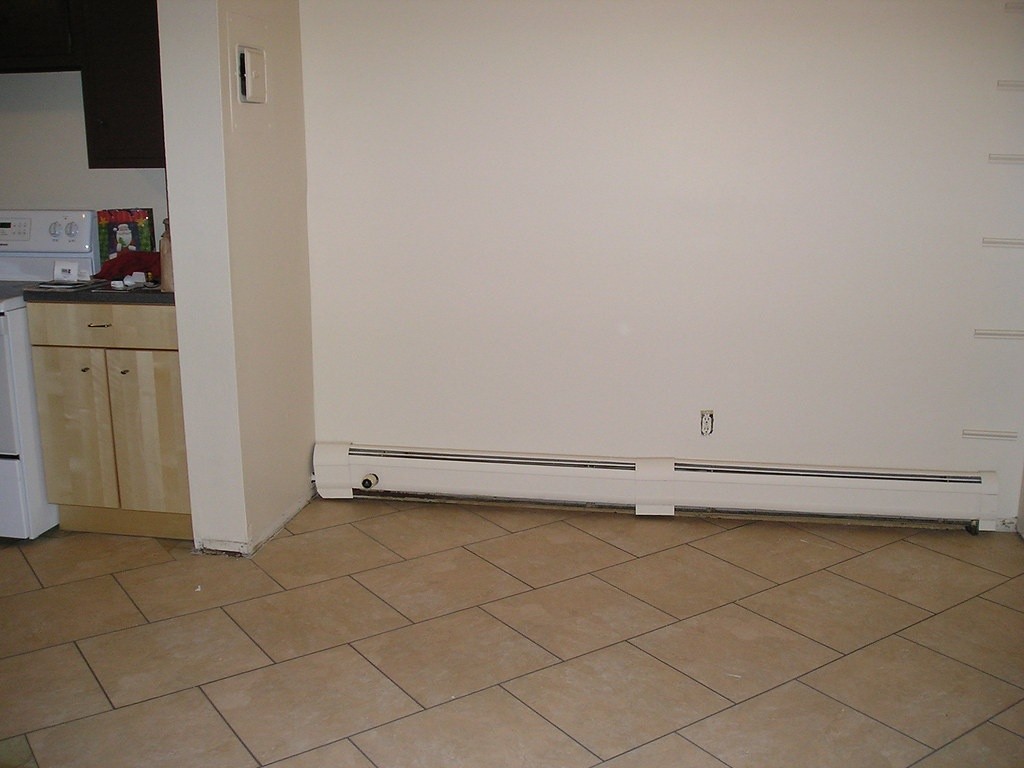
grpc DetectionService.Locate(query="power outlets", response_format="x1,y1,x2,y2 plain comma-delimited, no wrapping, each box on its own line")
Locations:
700,410,714,436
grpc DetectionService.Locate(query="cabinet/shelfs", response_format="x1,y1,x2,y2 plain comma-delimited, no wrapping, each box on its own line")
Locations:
0,0,81,74
79,0,166,168
26,303,194,540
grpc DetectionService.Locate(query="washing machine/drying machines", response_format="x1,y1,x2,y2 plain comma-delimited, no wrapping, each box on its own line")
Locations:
0,211,99,540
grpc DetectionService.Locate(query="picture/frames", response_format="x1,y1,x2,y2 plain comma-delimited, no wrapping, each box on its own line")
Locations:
97,208,156,266
